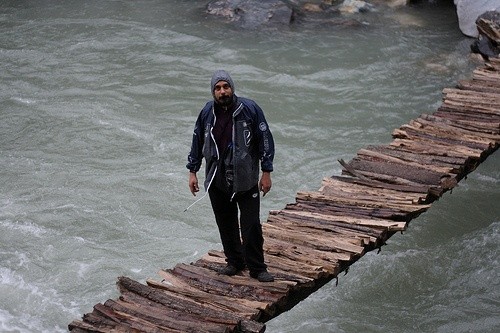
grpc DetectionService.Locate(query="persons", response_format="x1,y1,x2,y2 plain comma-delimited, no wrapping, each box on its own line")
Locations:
186,68,277,283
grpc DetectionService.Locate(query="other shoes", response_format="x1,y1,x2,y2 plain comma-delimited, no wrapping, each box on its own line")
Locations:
257,271,274,283
219,265,243,276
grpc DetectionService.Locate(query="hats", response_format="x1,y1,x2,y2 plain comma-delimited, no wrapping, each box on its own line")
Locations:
210,69,235,105
